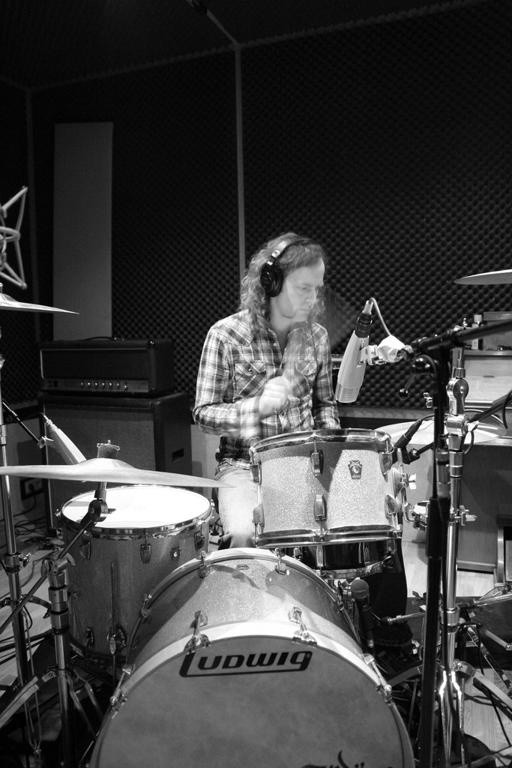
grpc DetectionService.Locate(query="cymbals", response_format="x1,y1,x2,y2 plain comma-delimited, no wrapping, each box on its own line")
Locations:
0,292,80,315
376,421,507,445
0,455,224,488
455,270,512,285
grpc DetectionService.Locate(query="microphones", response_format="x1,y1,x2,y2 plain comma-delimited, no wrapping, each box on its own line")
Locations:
335,300,377,405
351,579,376,653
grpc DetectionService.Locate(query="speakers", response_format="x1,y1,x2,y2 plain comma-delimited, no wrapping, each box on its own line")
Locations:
39,337,177,400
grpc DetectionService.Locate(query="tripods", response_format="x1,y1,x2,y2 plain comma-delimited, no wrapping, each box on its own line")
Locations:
0,547,102,768
387,342,511,768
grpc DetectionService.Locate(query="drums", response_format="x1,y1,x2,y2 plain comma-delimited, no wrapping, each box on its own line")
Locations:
284,537,397,582
247,428,406,547
87,548,415,767
54,484,212,665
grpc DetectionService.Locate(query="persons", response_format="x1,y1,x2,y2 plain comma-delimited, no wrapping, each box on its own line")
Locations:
191,232,341,548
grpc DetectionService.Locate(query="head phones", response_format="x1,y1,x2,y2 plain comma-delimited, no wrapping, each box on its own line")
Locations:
260,234,315,297
